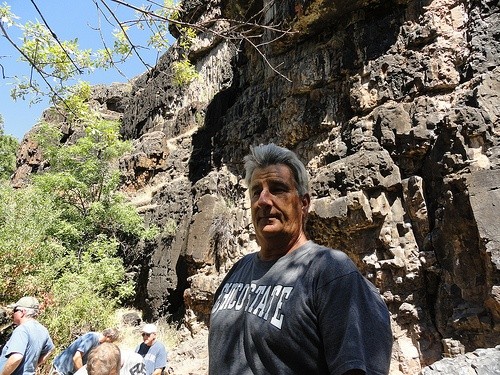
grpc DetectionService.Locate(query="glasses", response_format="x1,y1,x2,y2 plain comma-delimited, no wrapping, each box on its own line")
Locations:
14,307,26,314
142,333,154,337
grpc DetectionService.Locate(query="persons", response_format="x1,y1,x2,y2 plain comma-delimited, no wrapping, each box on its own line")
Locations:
208,144,392,375
136,325,167,375
1,296,55,375
48,328,119,375
72,342,146,375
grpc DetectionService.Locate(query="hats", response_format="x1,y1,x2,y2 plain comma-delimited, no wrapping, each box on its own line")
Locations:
7,297,39,309
142,324,157,333
